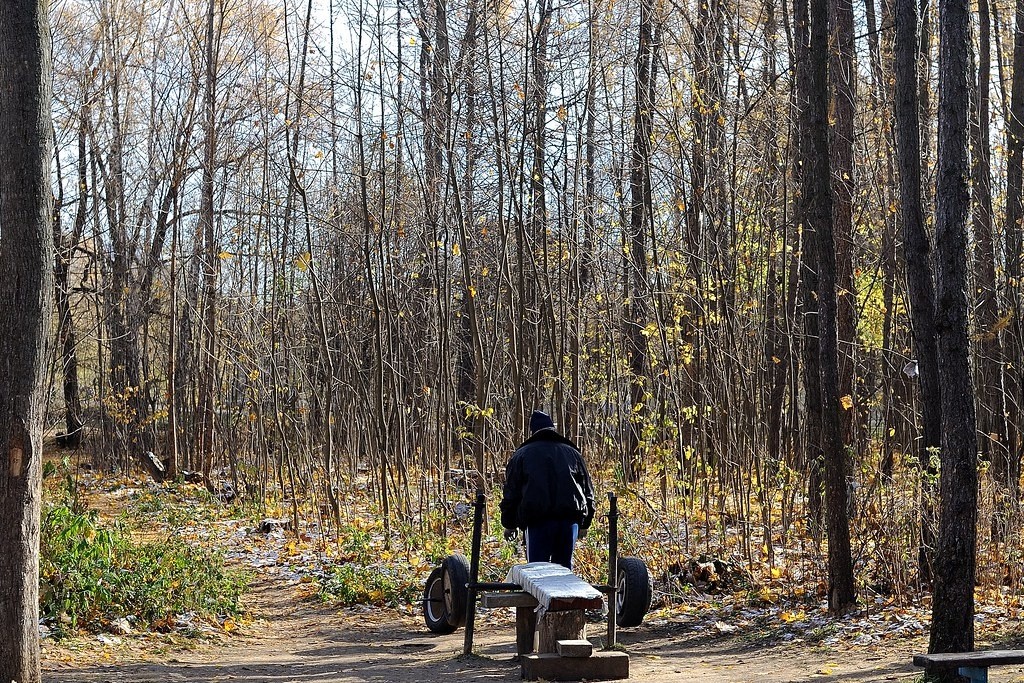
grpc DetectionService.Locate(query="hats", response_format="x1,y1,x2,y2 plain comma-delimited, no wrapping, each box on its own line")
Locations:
530,410,555,433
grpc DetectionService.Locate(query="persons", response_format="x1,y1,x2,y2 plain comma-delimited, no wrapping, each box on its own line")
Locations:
498,409,596,570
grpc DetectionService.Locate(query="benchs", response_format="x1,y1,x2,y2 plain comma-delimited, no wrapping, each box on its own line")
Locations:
913,650,1024,683
